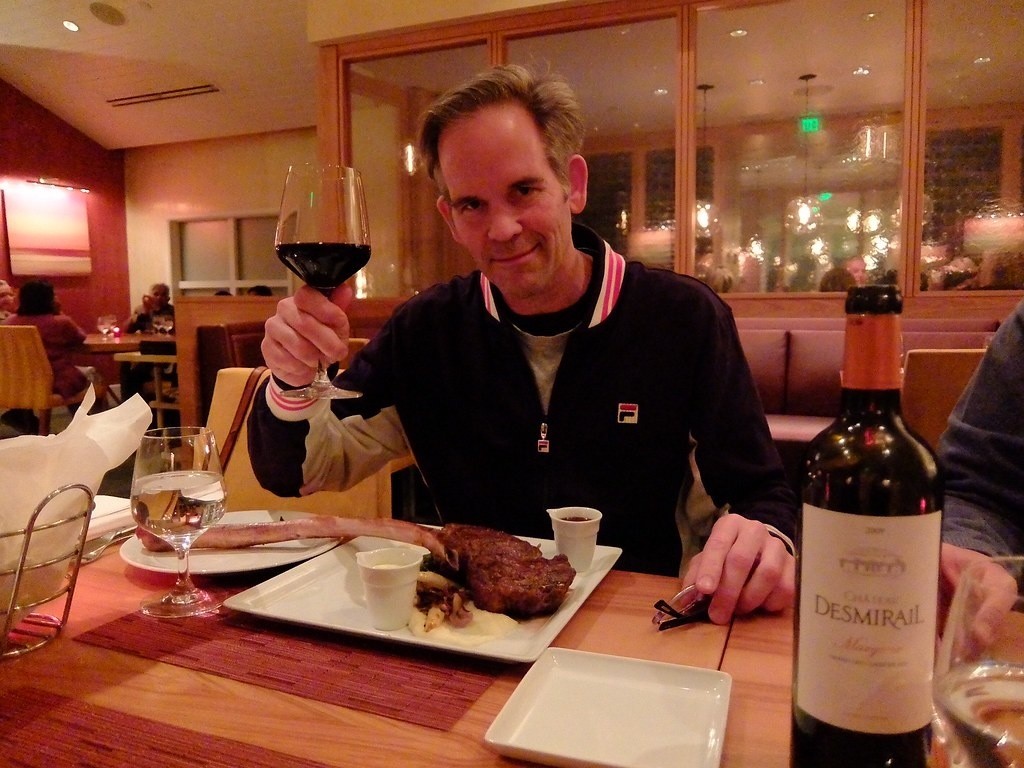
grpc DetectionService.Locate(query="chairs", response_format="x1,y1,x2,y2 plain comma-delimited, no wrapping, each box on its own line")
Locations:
0,326,104,437
187,364,395,520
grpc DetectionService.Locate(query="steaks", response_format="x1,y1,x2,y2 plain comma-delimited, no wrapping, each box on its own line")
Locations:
434,521,578,618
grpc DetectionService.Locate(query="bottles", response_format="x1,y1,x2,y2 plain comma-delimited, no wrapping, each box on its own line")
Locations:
789,285,945,768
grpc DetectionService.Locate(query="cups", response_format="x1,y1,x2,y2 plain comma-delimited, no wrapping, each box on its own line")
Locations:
355,548,423,632
932,556,1024,768
547,507,603,572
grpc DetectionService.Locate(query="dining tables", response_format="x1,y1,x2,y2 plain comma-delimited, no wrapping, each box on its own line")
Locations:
0,509,1024,768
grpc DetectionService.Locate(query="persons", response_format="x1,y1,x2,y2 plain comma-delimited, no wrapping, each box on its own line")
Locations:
248,286,273,298
119,283,176,419
213,289,235,298
1,280,21,323
932,301,1024,660
242,65,803,626
2,279,106,421
815,252,873,291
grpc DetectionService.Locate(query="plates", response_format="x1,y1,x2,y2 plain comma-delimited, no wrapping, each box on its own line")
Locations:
223,523,623,666
87,496,133,538
118,510,343,575
485,647,732,768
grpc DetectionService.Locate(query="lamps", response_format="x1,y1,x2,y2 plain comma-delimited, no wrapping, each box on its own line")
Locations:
685,80,915,284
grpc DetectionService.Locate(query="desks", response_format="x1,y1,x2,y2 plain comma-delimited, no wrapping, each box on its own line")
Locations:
116,351,180,431
78,330,178,358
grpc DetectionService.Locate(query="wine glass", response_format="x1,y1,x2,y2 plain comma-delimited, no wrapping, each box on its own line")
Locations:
152,315,165,337
130,426,228,619
273,165,370,401
107,314,117,339
163,320,173,337
96,315,110,341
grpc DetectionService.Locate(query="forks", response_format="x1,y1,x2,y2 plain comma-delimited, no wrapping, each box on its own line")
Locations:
80,533,134,565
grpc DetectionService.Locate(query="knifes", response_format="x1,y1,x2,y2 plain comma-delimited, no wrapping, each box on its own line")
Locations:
81,524,138,556
